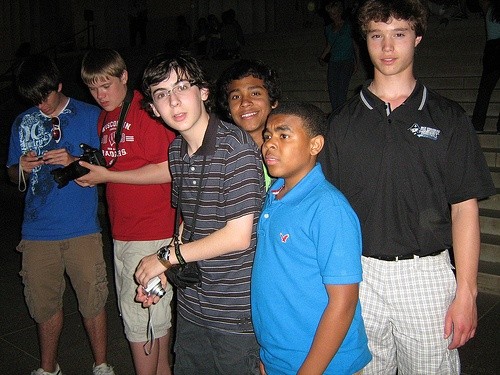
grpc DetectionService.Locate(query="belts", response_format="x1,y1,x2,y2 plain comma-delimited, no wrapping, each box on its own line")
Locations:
371,248,447,261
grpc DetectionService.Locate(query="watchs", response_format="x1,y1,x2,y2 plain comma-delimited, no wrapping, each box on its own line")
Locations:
156,245,173,269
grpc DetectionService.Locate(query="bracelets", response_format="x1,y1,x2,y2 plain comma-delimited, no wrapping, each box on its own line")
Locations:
175,243,188,267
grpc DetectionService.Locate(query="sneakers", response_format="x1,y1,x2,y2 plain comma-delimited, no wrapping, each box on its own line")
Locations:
31,363,62,375
92,361,114,375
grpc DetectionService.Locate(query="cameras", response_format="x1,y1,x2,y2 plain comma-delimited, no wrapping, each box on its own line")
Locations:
37,155,43,160
50,143,108,189
141,276,167,299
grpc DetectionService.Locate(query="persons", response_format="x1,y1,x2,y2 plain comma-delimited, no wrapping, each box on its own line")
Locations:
213,57,283,192
316,0,497,375
74,49,185,375
164,8,246,60
127,1,148,49
471,0,500,135
250,102,373,375
4,56,114,375
133,51,266,375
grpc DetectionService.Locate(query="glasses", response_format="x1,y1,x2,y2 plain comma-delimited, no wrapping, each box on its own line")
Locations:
51,114,61,143
152,81,197,104
